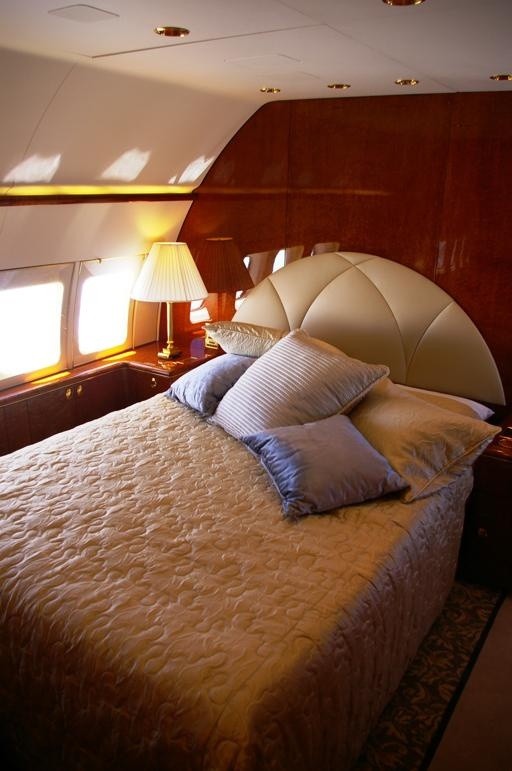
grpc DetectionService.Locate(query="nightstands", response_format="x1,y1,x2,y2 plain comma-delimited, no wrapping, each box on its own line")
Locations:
454,432,512,591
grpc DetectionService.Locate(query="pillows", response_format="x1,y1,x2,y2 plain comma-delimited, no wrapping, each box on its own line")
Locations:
234,411,412,520
392,380,495,420
164,353,258,416
216,337,390,443
345,376,501,501
199,318,347,356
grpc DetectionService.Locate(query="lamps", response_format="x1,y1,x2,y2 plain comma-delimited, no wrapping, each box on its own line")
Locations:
133,240,208,358
192,235,255,350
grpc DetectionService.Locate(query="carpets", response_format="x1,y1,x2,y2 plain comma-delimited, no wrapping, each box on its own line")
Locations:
348,559,512,770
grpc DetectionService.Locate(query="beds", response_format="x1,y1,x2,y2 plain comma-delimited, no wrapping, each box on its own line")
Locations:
0,251,511,768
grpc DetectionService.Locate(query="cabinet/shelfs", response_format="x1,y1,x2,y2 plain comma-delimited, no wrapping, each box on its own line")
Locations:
123,364,191,407
25,366,125,443
0,398,32,458
